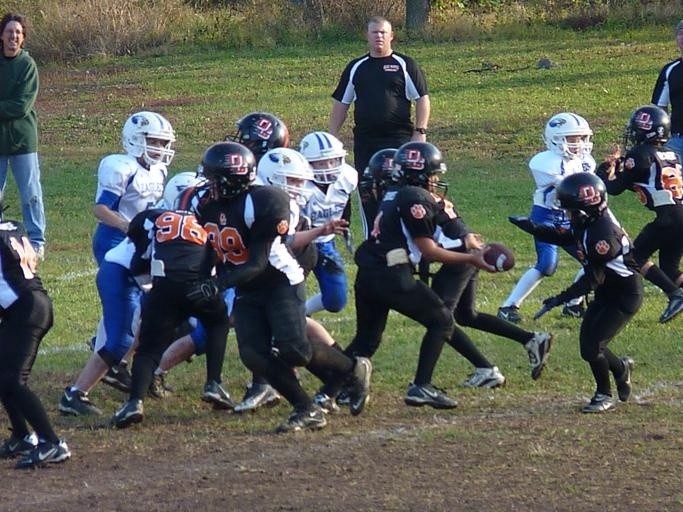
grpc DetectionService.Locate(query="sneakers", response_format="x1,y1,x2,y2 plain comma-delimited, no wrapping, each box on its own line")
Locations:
0,431,40,459
15,437,69,468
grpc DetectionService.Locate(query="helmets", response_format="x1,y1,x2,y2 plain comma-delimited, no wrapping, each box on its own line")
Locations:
541,106,672,227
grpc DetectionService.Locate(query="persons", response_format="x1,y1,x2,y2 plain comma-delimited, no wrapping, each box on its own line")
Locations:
651,19,683,166
507,170,644,413
0,11,47,260
597,104,683,323
497,110,597,327
0,191,71,468
57,111,553,437
329,16,431,237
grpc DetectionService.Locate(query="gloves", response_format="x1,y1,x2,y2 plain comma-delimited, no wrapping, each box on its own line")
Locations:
508,216,537,236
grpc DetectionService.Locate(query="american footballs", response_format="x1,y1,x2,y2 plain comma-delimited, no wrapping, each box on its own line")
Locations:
484,243,514,271
